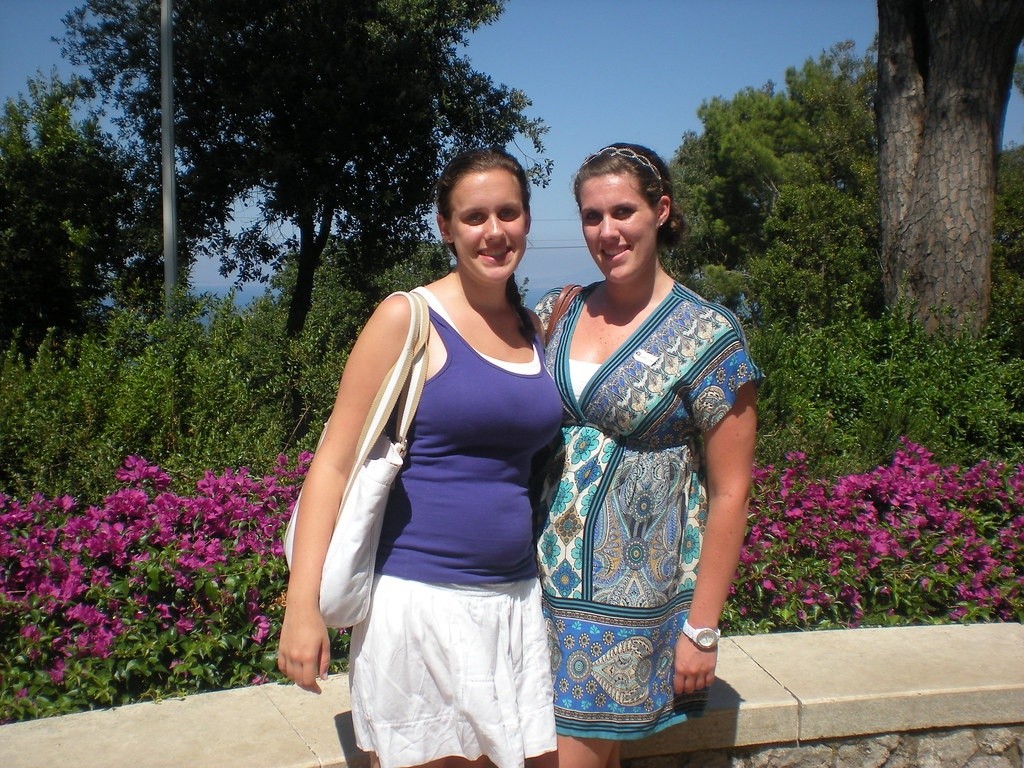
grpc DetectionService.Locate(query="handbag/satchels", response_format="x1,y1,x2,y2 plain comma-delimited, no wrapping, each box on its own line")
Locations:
282,289,435,630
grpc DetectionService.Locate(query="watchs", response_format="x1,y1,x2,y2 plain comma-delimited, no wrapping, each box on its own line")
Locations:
682,619,721,649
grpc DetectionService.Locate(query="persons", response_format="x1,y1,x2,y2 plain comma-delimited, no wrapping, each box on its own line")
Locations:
534,142,768,768
278,149,564,768
